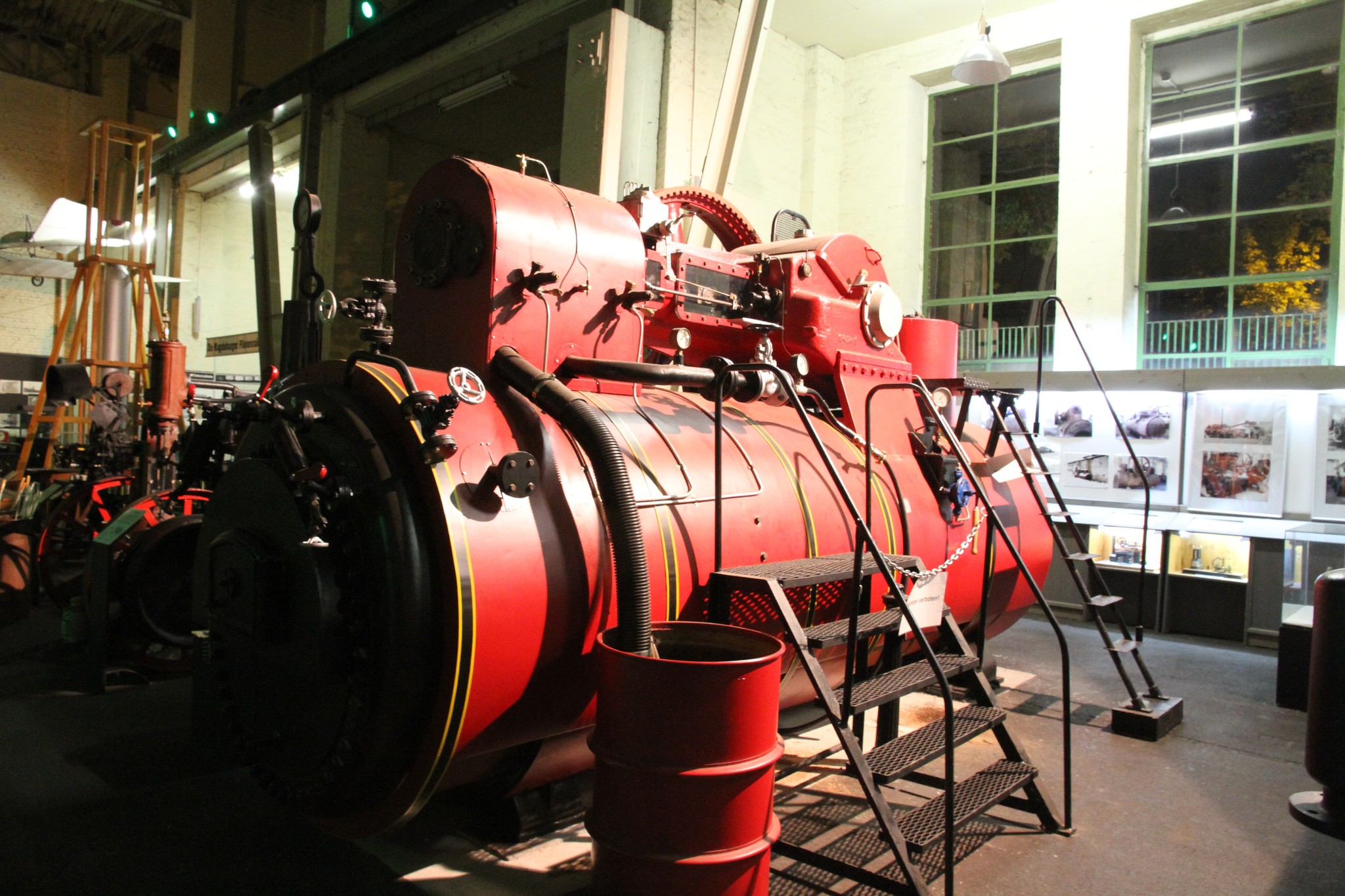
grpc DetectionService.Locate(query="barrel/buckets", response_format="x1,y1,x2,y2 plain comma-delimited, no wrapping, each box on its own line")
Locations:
581,620,788,895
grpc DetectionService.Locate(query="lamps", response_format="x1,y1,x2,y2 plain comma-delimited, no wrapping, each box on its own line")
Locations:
1160,110,1195,230
951,7,1012,84
1151,103,1256,141
